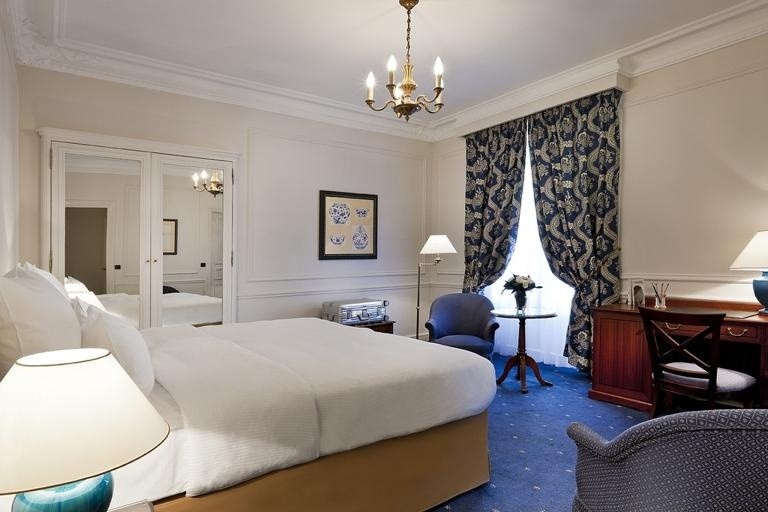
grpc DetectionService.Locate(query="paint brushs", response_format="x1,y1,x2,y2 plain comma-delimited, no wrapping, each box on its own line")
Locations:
652,282,670,304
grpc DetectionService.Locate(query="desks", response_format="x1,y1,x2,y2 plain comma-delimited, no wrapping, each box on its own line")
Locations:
491,309,557,393
588,301,768,412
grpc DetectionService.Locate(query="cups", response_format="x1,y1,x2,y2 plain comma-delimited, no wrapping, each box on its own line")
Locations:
654,295,667,311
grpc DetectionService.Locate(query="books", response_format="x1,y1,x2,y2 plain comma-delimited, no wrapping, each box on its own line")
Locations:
628,279,645,306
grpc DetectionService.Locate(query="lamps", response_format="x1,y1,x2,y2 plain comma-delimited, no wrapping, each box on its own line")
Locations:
415,233,458,339
191,169,224,198
729,231,768,317
1,349,172,512
366,0,445,125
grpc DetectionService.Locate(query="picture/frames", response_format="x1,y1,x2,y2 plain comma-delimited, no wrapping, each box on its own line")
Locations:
318,190,379,262
162,217,178,255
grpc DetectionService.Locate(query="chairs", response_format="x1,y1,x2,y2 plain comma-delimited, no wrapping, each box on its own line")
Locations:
638,305,757,419
425,293,501,362
566,408,768,512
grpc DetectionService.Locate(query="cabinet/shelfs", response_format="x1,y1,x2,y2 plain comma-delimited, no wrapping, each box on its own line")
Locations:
357,320,394,335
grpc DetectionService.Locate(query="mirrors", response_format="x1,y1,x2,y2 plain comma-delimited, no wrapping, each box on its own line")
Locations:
64,152,142,330
159,163,222,328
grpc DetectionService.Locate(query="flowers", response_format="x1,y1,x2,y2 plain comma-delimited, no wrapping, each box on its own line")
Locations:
502,273,543,305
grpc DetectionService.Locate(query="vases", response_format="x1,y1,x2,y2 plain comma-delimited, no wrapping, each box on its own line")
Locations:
515,294,527,313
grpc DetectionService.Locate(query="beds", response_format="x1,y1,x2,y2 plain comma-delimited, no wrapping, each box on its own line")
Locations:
96,293,222,326
1,260,496,512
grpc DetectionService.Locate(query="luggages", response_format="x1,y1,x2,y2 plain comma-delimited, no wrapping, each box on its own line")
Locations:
322,298,389,326
